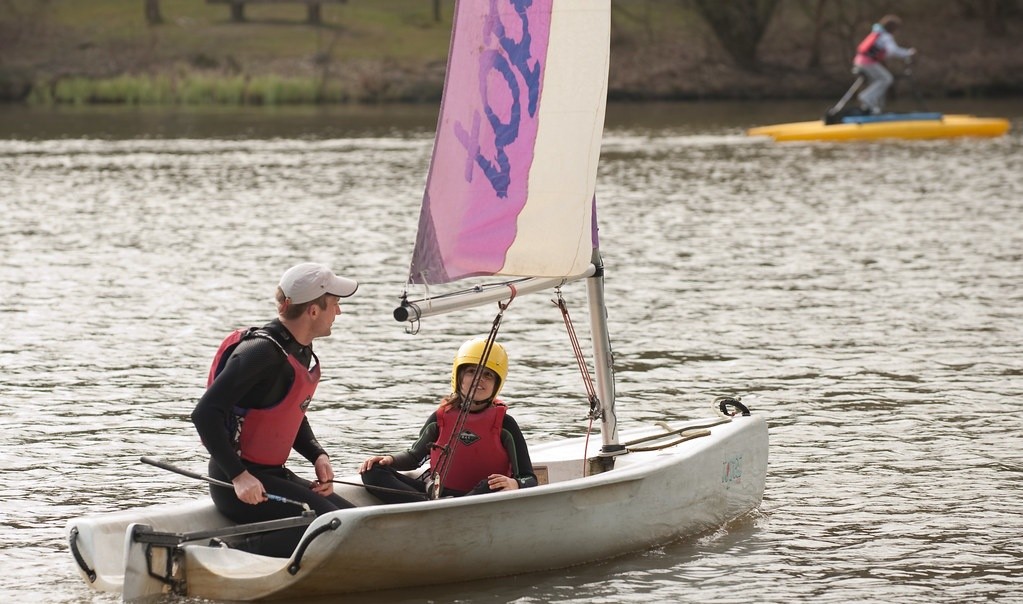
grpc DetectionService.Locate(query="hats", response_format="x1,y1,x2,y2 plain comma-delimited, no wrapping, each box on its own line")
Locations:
277,262,360,308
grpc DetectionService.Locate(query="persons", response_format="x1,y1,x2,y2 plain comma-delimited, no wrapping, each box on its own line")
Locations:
850,13,918,116
187,260,365,560
357,334,539,512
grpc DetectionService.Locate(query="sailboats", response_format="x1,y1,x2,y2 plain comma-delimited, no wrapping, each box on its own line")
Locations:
68,3,767,601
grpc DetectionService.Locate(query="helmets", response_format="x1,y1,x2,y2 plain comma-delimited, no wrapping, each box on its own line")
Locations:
454,335,509,380
